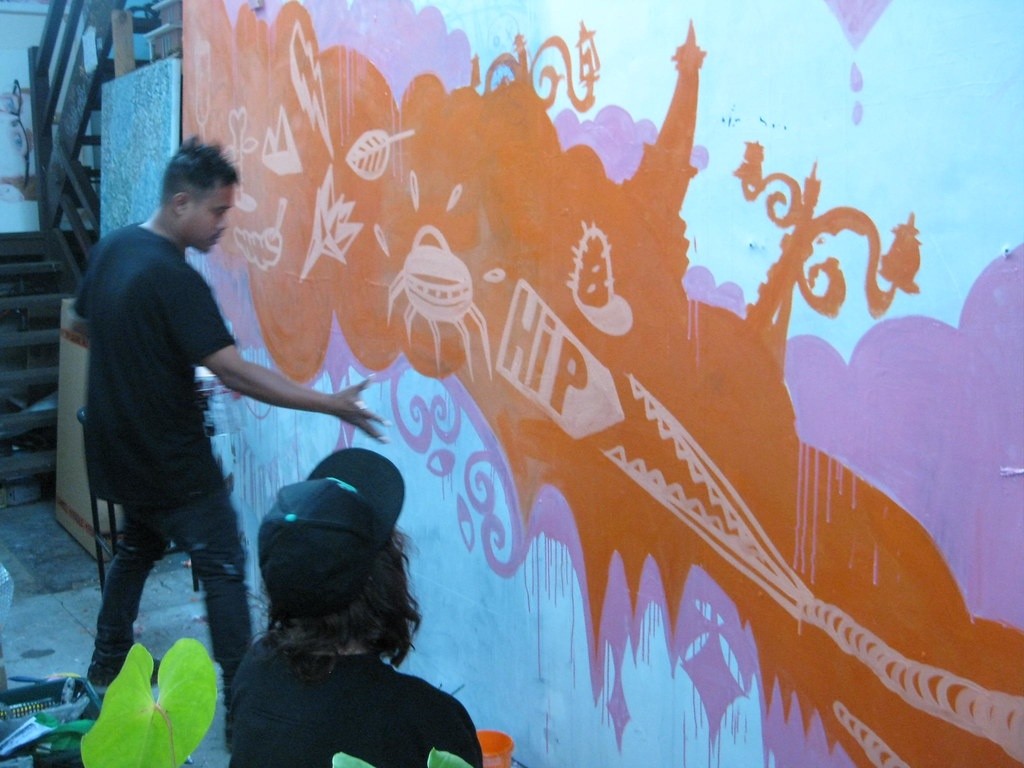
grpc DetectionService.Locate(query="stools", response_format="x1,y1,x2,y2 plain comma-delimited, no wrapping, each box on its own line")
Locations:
76,407,201,592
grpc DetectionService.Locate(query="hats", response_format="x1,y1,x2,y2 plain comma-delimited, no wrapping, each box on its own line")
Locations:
258,448,406,620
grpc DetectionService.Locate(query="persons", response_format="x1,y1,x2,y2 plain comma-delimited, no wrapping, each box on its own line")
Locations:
226,448,484,768
72,134,391,695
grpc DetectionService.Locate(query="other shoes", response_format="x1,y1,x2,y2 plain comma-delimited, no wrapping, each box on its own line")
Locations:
87,655,160,687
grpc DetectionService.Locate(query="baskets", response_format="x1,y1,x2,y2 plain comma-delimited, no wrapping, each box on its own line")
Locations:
0,677,101,721
